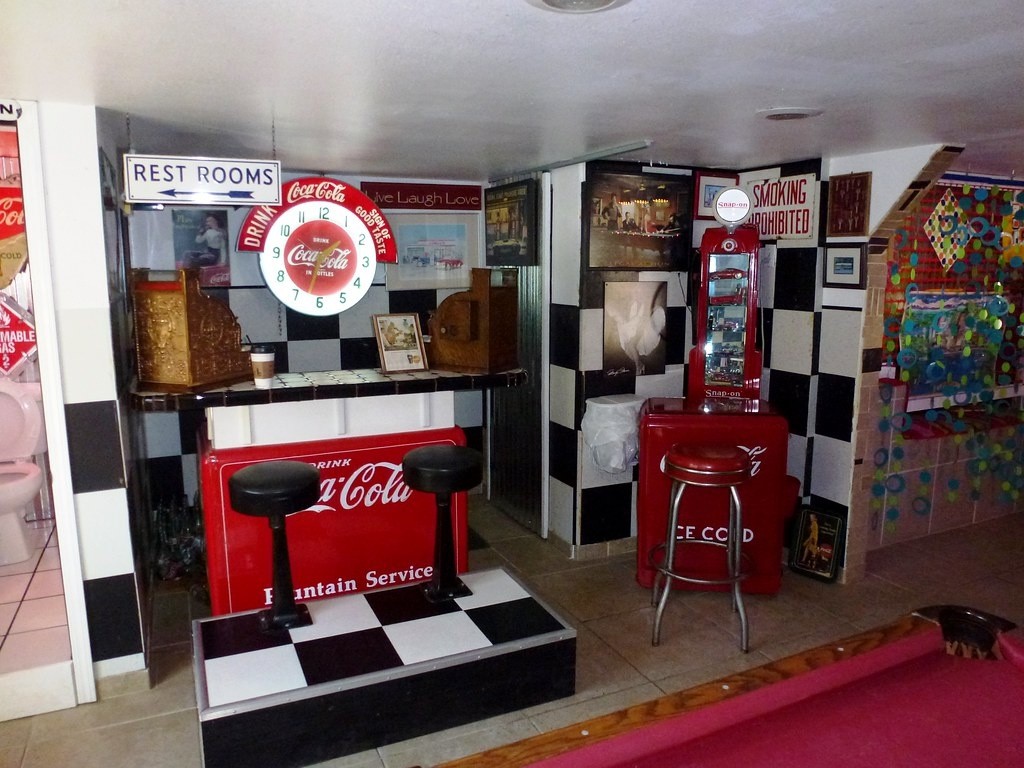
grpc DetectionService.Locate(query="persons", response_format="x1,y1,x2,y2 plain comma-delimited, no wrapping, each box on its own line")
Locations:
600,191,688,235
800,514,822,567
617,283,667,375
184,212,228,280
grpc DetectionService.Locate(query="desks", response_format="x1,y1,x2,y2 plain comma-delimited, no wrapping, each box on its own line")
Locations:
411,605,1024,768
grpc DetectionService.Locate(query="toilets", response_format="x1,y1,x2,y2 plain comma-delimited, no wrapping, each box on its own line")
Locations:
0,383,49,567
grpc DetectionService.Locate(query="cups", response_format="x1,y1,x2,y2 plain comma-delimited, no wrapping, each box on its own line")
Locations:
249,343,276,391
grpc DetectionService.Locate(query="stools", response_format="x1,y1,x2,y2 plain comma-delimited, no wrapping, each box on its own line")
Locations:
229,460,321,630
403,445,484,603
651,443,749,654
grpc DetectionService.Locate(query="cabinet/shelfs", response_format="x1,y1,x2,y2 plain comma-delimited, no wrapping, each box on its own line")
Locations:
635,398,788,593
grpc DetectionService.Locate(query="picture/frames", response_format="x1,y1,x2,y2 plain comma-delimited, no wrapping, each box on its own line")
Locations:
582,159,693,275
380,209,482,292
822,243,868,290
826,171,872,237
371,313,429,374
789,507,846,584
484,178,537,265
693,168,739,220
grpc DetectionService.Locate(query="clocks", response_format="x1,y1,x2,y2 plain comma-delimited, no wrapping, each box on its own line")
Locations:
234,176,399,317
